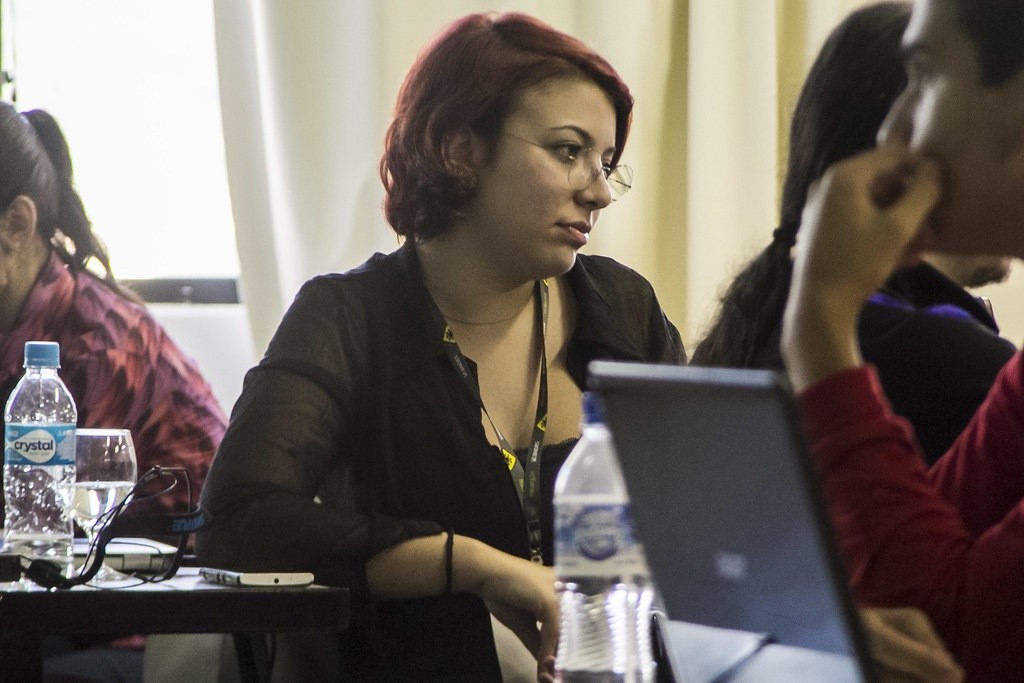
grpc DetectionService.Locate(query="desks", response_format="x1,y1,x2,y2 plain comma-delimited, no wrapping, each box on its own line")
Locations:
0,565,351,683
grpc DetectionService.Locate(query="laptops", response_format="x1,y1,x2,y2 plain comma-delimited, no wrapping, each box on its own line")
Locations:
590,360,881,683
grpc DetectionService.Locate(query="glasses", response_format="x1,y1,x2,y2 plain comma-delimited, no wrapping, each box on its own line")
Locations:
497,128,635,201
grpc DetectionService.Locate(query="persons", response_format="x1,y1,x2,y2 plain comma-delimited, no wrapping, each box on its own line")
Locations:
689,0,1015,473
194,8,688,683
0,99,227,683
780,0,1024,682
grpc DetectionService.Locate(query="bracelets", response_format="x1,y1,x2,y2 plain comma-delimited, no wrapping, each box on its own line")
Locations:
442,527,453,595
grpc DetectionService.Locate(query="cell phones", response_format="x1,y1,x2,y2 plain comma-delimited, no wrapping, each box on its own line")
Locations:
199,567,314,587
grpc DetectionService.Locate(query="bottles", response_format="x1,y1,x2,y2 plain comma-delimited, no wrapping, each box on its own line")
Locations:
3,340,78,590
552,393,658,683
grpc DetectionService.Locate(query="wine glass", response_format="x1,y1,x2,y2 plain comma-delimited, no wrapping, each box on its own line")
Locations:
74,428,143,587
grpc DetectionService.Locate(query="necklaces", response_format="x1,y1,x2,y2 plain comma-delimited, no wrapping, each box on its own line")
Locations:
441,286,535,325
